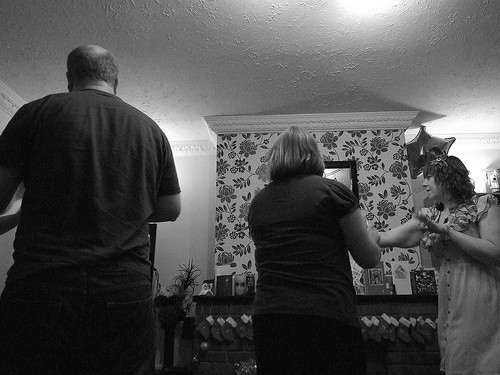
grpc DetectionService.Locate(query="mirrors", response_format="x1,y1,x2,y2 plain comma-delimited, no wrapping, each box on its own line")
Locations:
321,160,359,204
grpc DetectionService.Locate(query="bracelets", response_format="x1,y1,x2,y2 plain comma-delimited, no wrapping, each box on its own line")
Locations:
444,226,451,241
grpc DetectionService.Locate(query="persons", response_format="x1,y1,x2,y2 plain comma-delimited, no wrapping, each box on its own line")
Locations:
234,275,246,296
247,126,381,375
376,156,500,375
0,45,181,375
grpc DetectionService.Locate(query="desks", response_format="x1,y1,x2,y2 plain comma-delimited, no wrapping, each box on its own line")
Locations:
191,295,441,375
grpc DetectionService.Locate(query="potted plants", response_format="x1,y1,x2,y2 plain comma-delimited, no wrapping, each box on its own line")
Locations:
154,256,202,370
